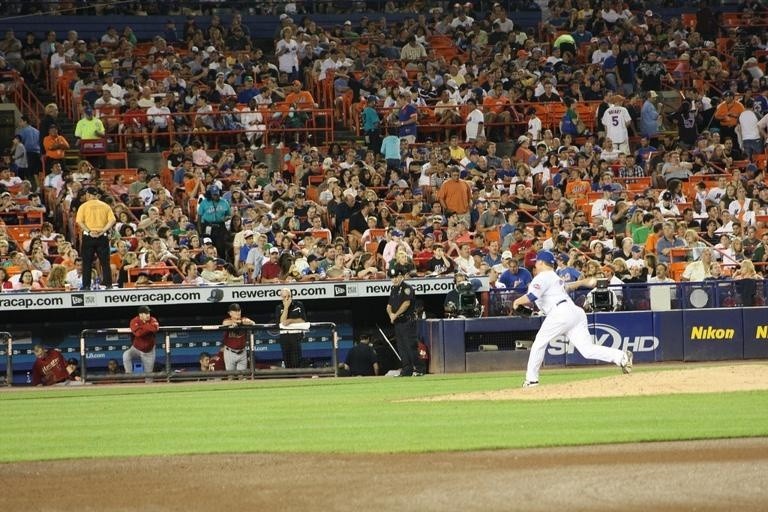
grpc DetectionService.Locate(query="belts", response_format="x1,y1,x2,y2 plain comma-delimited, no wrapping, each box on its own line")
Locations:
557,300,566,305
226,348,243,354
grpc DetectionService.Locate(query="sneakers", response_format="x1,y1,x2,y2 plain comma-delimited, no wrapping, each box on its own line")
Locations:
620,350,634,374
522,380,539,388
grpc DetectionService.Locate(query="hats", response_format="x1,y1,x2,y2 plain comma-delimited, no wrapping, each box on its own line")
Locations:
391,188,443,240
471,249,486,256
386,267,405,277
163,142,344,263
612,95,625,103
723,91,734,97
170,43,252,89
137,305,151,313
603,185,671,201
645,91,659,99
228,303,240,312
529,249,556,264
68,358,78,365
556,253,570,263
516,46,555,73
501,250,513,260
517,132,602,154
84,186,98,195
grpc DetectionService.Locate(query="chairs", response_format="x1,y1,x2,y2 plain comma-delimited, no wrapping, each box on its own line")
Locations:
0,1,768,315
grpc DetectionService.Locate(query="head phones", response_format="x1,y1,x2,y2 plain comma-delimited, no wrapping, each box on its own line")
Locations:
453,272,468,284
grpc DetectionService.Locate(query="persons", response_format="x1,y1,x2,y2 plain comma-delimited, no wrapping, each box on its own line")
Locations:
1,0,768,390
